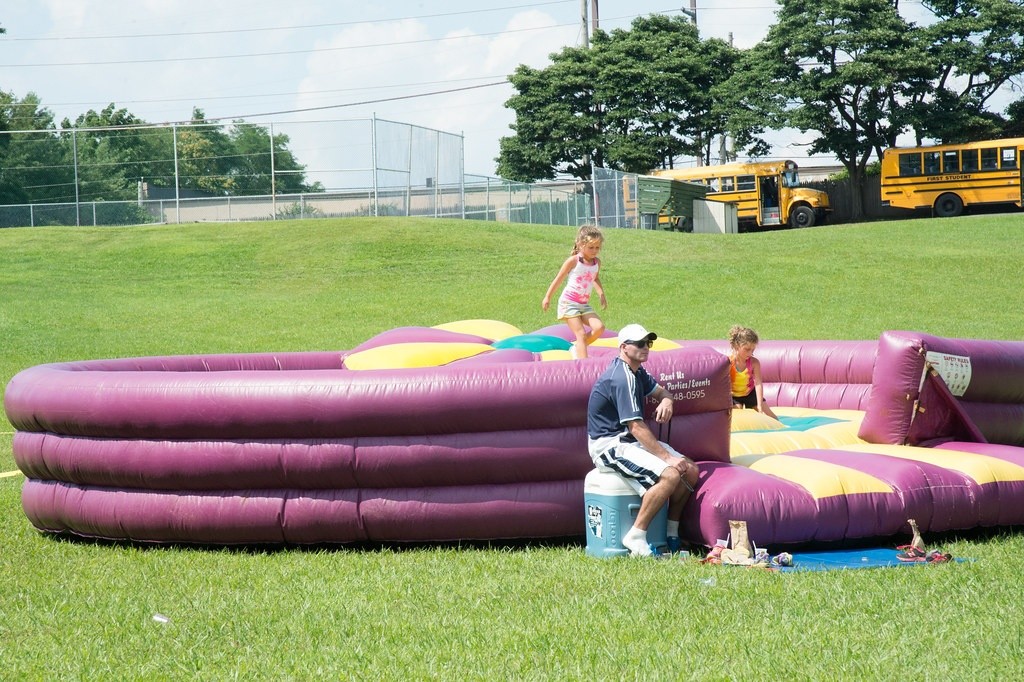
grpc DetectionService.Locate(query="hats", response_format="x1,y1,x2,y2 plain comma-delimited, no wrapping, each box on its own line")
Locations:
618,324,657,349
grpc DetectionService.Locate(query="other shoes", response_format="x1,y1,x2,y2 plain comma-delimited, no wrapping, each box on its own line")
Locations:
666,535,685,554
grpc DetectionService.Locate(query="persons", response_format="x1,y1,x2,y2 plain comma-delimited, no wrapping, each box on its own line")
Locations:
729,326,777,420
588,323,699,556
542,226,607,359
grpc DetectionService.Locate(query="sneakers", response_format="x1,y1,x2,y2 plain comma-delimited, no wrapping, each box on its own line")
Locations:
895,545,926,562
758,552,769,562
927,551,952,562
772,552,793,566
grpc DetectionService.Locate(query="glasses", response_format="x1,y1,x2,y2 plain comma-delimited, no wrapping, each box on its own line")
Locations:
679,472,694,492
624,340,654,349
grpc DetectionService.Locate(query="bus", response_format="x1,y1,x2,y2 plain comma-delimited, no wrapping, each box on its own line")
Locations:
880,137,1024,218
624,159,835,233
